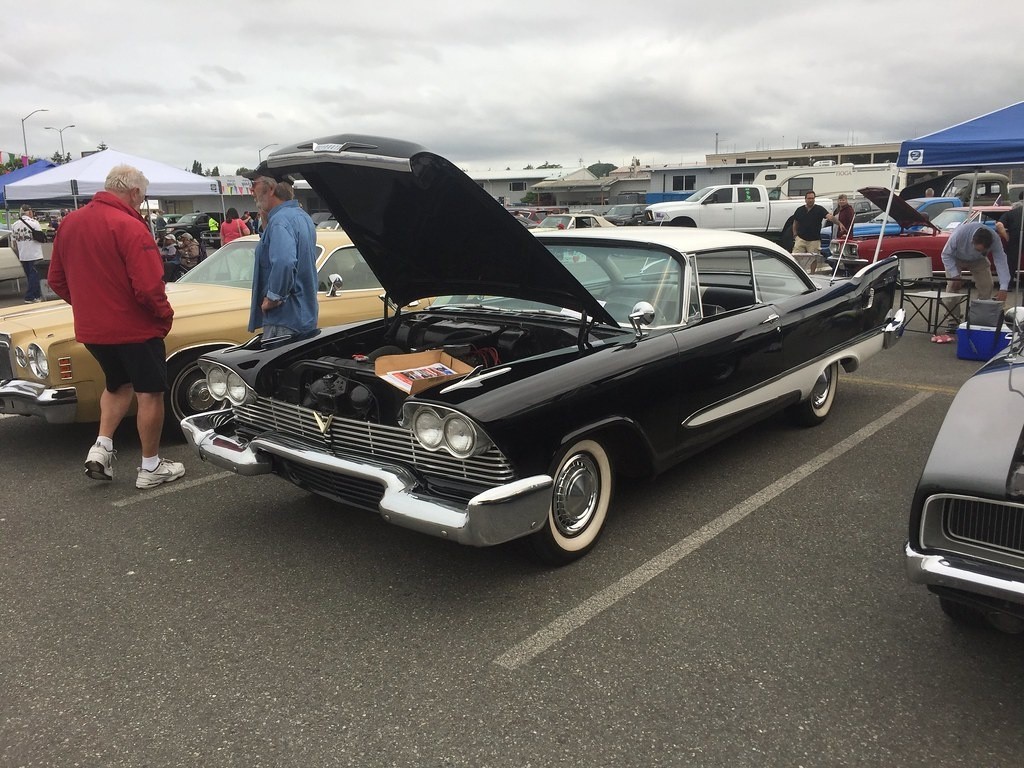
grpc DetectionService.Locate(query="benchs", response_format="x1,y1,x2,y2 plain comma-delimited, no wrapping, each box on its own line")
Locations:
602,285,756,311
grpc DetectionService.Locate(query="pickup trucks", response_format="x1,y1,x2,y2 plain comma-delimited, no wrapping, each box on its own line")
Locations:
941,172,1024,207
819,197,963,267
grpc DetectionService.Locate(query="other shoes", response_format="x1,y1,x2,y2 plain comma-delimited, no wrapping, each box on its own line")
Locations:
25,298,43,303
997,286,1011,292
945,324,958,334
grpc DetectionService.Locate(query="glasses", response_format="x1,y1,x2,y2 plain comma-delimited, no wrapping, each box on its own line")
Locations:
249,179,270,189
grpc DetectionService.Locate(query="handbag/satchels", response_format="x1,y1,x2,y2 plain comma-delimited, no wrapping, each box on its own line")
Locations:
29,230,46,244
968,300,1003,327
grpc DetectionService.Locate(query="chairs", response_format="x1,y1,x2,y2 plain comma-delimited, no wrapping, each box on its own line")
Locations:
711,194,719,203
898,257,971,337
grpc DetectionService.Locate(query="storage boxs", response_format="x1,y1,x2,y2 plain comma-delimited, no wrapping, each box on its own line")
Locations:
375,351,475,395
957,322,1013,362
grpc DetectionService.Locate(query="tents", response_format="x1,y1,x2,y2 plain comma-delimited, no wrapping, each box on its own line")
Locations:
0,148,220,210
872,101,1024,263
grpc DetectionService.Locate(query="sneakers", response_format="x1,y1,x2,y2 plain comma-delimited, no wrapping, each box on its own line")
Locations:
135,457,185,489
83,441,117,481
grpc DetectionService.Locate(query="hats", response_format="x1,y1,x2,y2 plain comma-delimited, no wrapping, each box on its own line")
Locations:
21,204,31,211
165,234,176,242
1019,191,1024,200
241,159,294,185
180,233,193,240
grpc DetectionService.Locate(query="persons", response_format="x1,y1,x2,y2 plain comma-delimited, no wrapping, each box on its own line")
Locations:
48,165,185,489
941,191,1024,333
237,159,319,338
789,191,856,276
10,204,44,303
144,208,263,282
925,188,934,197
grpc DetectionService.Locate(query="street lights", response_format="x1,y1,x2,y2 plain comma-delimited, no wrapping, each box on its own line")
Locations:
21,109,49,157
258,144,279,165
44,124,76,165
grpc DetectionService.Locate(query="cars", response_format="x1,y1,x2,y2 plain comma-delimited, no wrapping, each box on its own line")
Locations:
903,299,1024,633
644,183,834,234
162,211,348,248
504,204,652,233
9,207,63,222
0,230,438,426
180,133,906,580
826,185,1024,281
